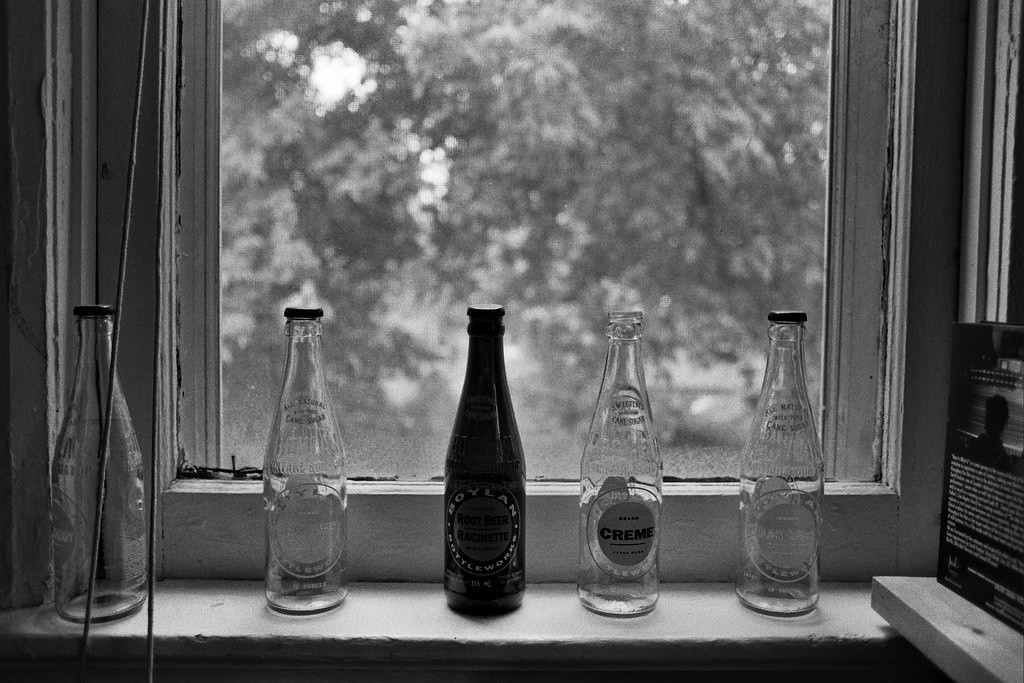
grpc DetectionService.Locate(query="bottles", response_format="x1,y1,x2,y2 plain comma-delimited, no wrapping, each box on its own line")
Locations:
54,305,146,628
266,307,350,620
443,303,527,618
734,308,822,616
580,310,662,616
